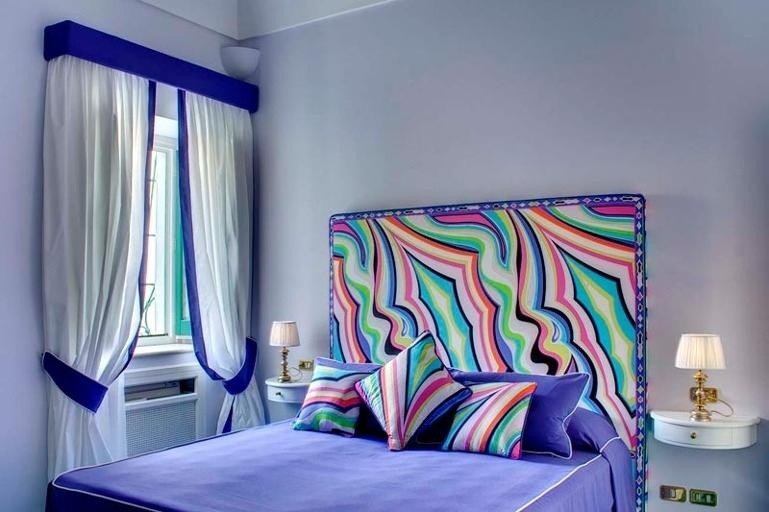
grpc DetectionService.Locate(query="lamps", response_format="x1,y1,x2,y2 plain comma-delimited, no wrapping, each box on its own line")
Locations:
675,334,726,422
270,321,300,382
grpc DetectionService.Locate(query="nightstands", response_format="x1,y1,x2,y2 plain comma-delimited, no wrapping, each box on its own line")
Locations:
651,409,759,451
265,376,310,404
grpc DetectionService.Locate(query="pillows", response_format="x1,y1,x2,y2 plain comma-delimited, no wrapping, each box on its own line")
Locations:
441,379,539,460
292,356,383,438
448,369,590,461
354,328,474,451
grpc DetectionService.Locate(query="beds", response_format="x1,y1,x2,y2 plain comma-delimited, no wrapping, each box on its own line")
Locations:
47,193,646,511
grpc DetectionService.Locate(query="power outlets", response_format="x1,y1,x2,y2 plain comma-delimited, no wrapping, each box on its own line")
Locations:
691,388,716,402
299,360,313,370
